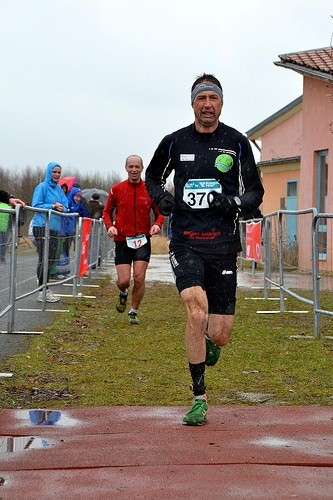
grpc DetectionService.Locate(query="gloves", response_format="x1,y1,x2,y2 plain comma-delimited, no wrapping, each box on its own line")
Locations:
159,192,184,216
214,194,237,216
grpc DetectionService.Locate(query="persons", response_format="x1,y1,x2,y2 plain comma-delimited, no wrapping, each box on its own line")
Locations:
144,73,266,425
102,155,165,325
0,188,26,262
30,162,90,303
87,194,107,270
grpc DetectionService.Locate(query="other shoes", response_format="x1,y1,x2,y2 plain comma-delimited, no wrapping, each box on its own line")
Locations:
58,275,65,279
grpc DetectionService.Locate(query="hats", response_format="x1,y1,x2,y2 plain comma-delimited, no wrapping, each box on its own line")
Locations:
75,192,82,197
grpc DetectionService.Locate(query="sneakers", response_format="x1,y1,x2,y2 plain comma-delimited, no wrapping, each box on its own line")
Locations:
205,335,221,366
182,399,209,426
37,289,61,303
116,292,128,312
128,311,139,324
